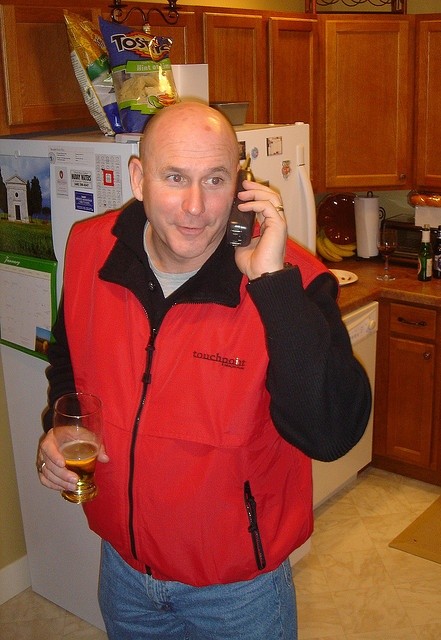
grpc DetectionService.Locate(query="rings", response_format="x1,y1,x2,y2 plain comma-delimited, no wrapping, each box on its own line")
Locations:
274,203,286,213
33,459,49,474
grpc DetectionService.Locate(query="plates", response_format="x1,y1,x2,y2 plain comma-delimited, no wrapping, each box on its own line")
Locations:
328,269,359,286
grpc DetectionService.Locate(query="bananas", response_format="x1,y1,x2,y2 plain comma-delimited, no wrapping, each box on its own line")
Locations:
317,229,357,262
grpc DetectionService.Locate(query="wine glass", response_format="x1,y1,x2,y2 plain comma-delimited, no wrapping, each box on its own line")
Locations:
376,228,398,281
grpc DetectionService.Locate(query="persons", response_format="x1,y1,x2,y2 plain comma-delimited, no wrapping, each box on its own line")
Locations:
33,95,371,636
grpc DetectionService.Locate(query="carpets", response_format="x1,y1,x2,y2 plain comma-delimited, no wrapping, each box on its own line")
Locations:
388,494,439,565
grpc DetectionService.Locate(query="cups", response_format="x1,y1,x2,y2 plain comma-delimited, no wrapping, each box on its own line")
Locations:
52,392,103,504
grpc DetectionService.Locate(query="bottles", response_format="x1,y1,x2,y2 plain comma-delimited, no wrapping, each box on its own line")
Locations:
434,225,441,279
417,224,434,281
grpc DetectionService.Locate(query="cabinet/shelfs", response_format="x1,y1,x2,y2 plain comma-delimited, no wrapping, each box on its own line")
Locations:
325,13,413,187
201,5,265,127
414,15,440,194
1,1,201,135
381,291,434,490
267,8,321,187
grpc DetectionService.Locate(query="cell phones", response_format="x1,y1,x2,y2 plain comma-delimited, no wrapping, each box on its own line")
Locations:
227,158,256,247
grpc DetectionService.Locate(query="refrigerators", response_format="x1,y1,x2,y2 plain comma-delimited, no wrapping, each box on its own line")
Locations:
1,120,313,634
